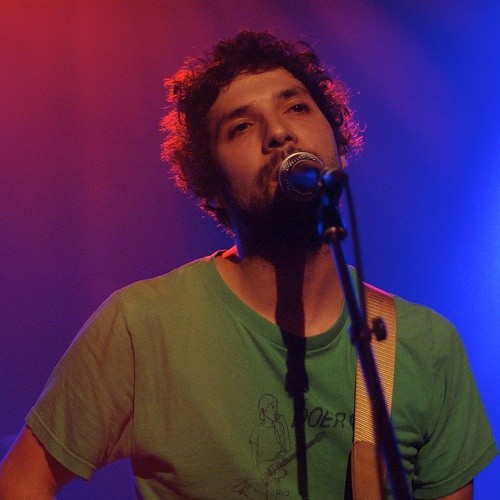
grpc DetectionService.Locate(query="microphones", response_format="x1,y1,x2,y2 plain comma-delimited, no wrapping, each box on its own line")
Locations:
280,152,349,203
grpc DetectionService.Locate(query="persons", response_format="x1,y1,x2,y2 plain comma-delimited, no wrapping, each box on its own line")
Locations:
0,28,499,499
